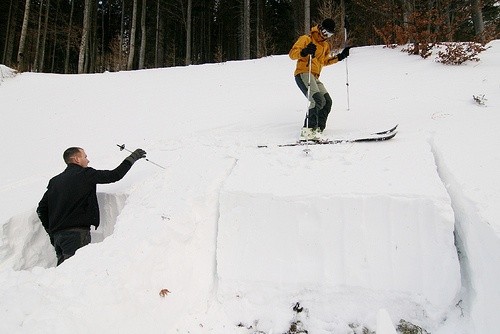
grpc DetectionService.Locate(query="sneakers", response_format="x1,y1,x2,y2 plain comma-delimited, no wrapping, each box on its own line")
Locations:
299,127,328,142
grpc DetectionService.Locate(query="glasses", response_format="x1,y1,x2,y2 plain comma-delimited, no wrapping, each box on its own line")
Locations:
321,25,334,38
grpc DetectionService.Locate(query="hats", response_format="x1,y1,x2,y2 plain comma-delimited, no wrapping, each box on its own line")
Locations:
322,18,335,33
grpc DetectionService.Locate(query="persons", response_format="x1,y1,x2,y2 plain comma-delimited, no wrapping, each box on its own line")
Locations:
36,147,147,266
289,18,349,143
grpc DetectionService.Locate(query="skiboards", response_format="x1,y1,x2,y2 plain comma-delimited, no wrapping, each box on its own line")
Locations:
257,124,399,148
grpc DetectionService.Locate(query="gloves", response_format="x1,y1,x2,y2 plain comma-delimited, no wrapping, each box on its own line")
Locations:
300,42,317,57
126,149,146,165
337,46,351,61
